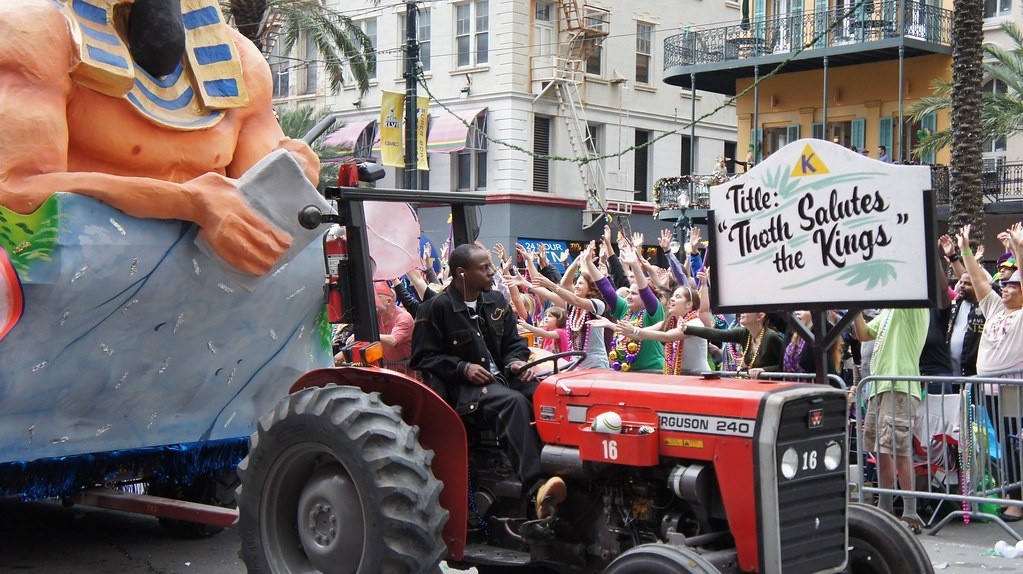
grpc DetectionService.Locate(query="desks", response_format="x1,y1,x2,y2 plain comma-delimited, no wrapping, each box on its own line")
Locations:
727,38,765,58
851,20,892,43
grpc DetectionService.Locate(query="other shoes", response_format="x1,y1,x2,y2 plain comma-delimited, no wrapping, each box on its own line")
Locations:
1000,506,1023,522
535,476,567,519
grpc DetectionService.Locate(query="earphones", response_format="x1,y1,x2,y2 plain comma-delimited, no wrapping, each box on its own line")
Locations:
460,272,465,279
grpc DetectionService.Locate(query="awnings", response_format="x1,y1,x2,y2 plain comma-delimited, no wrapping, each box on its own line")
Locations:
322,119,376,163
370,114,406,162
426,106,489,156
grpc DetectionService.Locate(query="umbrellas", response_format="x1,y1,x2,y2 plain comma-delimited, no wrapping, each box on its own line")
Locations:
864,0,875,13
740,0,751,30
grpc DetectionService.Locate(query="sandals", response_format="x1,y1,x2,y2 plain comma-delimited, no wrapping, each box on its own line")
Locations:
900,516,922,535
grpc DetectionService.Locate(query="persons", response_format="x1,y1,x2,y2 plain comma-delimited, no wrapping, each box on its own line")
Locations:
331,219,1023,535
1,1,323,279
850,145,858,152
409,243,567,520
877,145,889,163
716,161,728,174
728,152,754,173
862,149,869,157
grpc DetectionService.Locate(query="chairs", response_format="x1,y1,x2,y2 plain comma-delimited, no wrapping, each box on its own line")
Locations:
864,392,973,531
751,29,780,57
696,32,724,63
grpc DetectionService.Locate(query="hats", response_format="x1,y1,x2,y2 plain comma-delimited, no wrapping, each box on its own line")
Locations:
996,254,1018,270
1001,270,1023,289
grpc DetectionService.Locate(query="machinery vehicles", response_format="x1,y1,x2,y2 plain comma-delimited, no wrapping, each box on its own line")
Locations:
235,160,935,573
